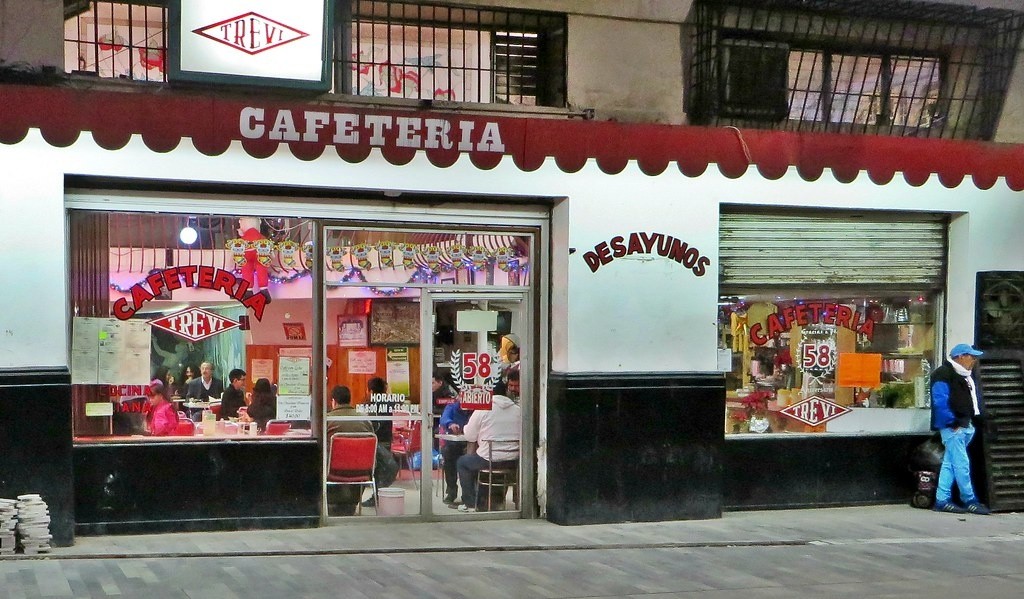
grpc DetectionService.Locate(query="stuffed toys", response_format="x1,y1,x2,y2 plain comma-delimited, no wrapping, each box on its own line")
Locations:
236,218,271,291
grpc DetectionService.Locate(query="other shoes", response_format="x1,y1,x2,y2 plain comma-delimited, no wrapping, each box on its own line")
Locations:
362,494,378,507
444,490,457,505
457,503,479,513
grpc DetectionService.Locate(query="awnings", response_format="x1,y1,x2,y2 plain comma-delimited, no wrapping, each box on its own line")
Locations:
0,85,1024,191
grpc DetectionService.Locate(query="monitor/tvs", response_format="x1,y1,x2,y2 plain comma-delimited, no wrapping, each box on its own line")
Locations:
367,301,420,347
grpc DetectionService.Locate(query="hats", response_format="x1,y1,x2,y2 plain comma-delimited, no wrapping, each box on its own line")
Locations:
949,344,984,358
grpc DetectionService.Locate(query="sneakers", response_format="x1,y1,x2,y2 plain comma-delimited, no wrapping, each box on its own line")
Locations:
965,502,989,515
932,501,965,513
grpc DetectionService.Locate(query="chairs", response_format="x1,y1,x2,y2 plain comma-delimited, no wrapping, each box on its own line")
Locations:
326,432,378,515
208,401,222,421
435,426,446,501
265,419,292,435
392,410,419,489
475,439,519,511
173,418,195,437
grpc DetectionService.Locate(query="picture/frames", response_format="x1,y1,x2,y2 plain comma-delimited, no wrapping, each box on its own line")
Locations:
336,313,368,348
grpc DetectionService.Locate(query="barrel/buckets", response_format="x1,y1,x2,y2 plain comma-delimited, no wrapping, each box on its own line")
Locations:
377,488,405,515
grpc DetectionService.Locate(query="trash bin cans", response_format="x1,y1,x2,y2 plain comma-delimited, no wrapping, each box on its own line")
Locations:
378,487,405,517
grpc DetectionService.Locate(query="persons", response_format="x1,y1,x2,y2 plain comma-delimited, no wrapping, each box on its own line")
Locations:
439,403,471,503
326,385,376,516
143,378,180,435
187,361,223,413
152,365,176,408
506,369,520,406
499,338,520,383
355,377,392,507
457,377,522,513
247,378,278,431
222,368,247,418
177,364,201,398
432,373,458,451
929,343,992,515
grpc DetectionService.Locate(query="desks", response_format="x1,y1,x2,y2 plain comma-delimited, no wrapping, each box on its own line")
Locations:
173,399,185,409
183,403,209,418
436,434,477,502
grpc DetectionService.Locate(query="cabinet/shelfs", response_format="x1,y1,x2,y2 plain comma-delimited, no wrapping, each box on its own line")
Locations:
851,297,934,409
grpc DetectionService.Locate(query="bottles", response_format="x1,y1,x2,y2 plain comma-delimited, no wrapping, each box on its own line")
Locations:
218,416,225,435
202,404,216,436
250,422,258,436
